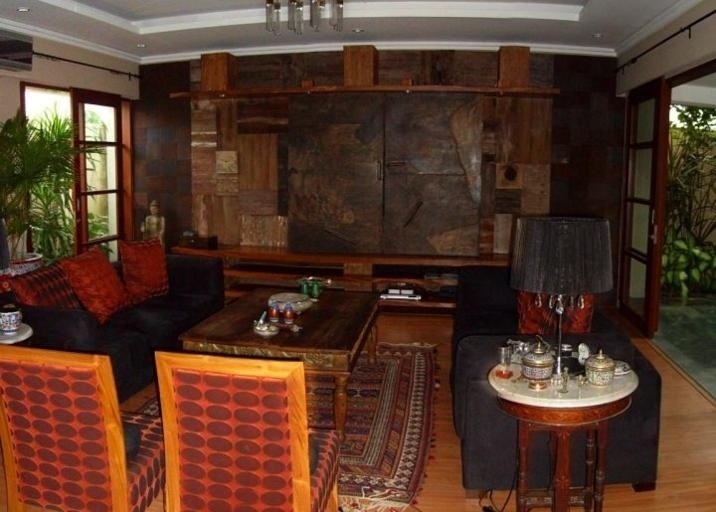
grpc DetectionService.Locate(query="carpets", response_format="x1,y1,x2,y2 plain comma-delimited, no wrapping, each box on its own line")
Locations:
132,341,442,511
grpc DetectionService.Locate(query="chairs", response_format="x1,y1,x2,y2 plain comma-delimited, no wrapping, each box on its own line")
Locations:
154,350,340,511
0,343,167,511
453,263,660,491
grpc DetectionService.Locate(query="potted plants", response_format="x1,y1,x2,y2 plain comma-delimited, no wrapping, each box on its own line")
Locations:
0,114,106,280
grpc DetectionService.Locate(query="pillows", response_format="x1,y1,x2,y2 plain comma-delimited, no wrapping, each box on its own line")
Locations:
8,238,168,321
514,286,597,339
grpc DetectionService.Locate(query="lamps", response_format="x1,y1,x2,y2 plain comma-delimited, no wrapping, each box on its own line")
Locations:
266,0,344,38
508,215,613,388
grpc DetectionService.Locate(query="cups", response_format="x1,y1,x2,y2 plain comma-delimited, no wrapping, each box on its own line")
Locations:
495,345,514,377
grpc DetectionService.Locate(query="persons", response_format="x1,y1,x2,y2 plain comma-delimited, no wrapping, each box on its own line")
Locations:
141,199,166,248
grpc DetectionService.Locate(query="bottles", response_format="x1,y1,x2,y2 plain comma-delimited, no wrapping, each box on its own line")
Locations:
283,302,295,324
268,299,280,323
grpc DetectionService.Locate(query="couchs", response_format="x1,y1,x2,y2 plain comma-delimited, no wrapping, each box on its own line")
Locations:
0,256,225,403
